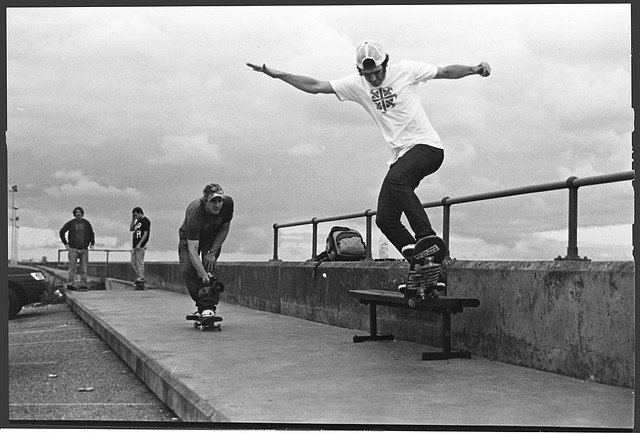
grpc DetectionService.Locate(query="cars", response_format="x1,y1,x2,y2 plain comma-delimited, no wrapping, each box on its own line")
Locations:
7,264,45,318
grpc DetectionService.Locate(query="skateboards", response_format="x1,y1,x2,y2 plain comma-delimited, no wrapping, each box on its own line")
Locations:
186,316,223,331
404,235,448,308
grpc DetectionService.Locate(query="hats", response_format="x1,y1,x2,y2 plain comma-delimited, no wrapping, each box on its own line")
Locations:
202,183,225,201
357,40,385,69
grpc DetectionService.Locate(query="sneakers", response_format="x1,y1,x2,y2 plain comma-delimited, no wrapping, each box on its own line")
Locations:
188,311,201,318
402,240,423,258
398,273,445,294
202,309,215,317
137,278,146,281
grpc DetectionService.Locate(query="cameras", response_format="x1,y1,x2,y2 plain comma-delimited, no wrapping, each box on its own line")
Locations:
195,277,225,307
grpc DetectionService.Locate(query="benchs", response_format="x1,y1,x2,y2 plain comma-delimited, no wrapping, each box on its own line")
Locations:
347,289,480,361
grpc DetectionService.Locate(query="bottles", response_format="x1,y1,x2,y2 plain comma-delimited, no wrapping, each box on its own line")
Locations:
380,234,388,260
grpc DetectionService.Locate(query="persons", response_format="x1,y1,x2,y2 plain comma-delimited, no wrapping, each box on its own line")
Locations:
178,182,234,317
246,40,491,294
59,206,95,288
130,206,150,282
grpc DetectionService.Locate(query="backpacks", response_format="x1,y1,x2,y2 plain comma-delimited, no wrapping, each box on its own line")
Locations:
312,225,367,277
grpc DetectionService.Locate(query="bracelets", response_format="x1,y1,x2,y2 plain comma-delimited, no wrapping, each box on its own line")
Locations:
478,63,483,73
207,249,215,254
